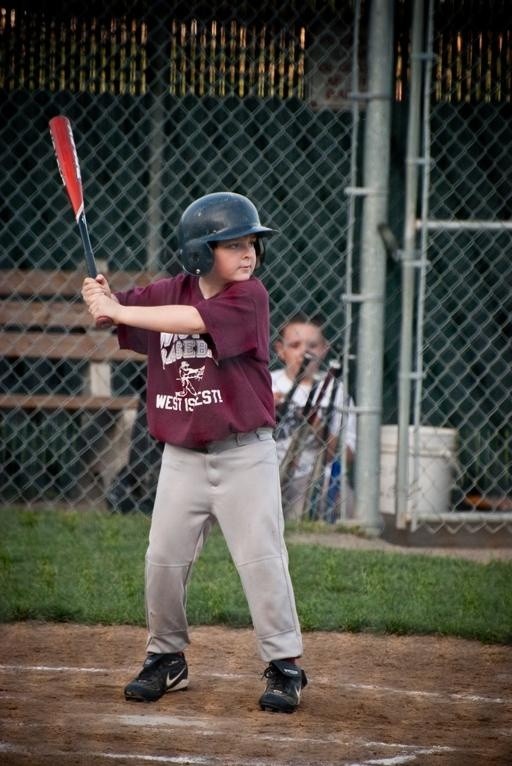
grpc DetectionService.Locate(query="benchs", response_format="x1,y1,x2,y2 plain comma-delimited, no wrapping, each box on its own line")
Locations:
0,259,174,506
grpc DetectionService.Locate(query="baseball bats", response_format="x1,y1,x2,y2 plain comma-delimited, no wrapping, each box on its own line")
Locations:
272,352,358,525
47,116,99,282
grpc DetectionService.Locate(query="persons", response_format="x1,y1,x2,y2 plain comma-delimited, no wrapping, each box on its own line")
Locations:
80,191,307,715
269,314,358,521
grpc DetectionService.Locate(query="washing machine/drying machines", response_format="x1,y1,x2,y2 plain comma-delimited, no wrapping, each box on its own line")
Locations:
378,424,456,517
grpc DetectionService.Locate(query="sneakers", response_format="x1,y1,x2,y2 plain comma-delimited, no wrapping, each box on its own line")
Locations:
124,651,190,703
257,660,307,714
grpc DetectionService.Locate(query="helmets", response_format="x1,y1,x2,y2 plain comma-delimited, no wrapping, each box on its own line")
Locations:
176,192,281,276
